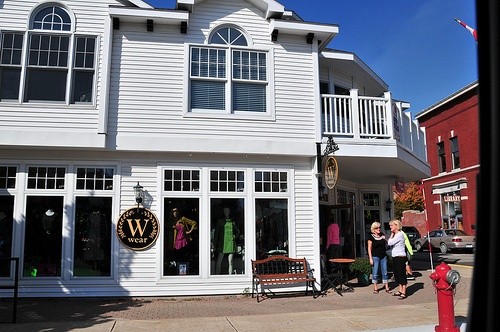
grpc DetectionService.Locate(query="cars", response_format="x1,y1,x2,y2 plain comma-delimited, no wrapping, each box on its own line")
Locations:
414,229,476,254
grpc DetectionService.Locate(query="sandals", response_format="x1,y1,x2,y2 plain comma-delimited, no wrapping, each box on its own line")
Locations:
398,293,407,299
392,291,402,296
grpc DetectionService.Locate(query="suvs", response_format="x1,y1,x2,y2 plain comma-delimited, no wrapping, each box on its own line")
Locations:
402,226,421,248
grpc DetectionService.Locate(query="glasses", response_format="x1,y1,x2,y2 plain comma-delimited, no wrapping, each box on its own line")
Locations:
373,227,381,229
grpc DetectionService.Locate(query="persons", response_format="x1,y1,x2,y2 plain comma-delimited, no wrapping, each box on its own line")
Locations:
216,208,242,273
389,230,415,281
368,222,392,294
172,208,198,275
326,219,340,273
388,219,409,299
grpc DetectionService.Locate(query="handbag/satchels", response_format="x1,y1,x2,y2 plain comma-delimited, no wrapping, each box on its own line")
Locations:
340,237,344,246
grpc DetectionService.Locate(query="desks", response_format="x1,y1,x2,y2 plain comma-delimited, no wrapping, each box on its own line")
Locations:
328,258,356,292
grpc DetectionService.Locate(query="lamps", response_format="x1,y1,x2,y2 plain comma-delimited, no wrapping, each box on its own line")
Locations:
384,198,392,210
134,181,144,204
320,185,326,194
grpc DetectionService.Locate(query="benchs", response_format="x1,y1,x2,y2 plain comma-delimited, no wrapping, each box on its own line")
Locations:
252,255,316,301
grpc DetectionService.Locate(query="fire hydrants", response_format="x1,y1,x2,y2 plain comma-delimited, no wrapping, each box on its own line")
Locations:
429,262,460,332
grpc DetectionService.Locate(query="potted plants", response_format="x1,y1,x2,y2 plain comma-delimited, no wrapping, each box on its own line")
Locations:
350,258,370,287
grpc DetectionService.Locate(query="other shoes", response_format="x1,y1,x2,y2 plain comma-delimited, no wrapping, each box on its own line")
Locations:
374,289,378,294
386,290,392,294
